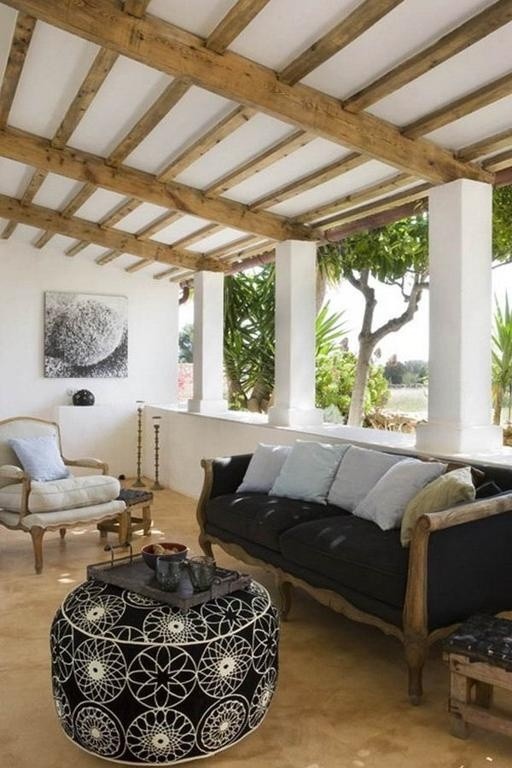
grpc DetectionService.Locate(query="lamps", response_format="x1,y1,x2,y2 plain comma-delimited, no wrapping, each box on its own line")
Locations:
133,401,146,487
150,417,164,491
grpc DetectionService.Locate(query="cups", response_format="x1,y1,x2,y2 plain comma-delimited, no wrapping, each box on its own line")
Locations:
157,557,184,592
189,557,217,593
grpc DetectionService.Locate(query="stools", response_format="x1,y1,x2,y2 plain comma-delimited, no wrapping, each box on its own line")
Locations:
441,614,512,740
97,489,154,544
49,580,279,766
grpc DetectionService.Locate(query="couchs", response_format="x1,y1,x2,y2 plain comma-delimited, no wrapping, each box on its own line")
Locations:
196,450,512,707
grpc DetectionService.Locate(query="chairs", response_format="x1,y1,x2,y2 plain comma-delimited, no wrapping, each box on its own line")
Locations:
0,416,127,574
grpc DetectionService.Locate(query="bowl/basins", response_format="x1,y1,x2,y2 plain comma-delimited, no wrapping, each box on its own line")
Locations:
140,540,187,577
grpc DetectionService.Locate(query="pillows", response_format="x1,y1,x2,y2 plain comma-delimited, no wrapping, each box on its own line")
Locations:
351,457,449,532
400,466,477,548
267,439,350,506
235,442,293,494
325,445,407,513
7,437,70,481
